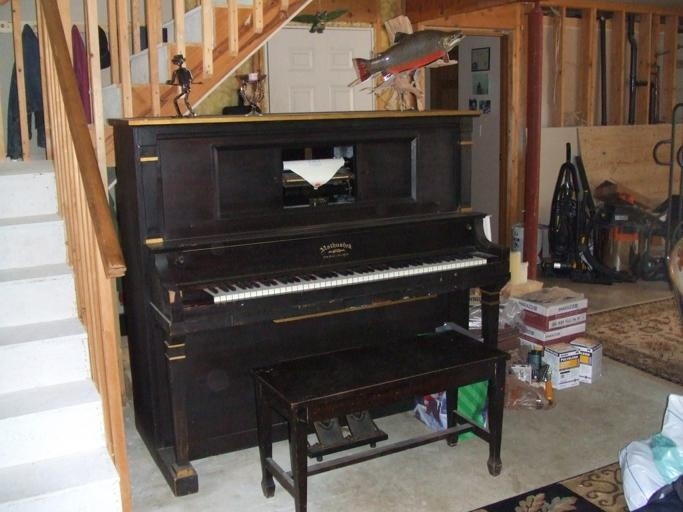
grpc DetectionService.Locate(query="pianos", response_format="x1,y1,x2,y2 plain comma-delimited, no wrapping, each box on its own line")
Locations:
105,109,511,496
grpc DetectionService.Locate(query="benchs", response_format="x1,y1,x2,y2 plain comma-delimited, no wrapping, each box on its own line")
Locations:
248,329,510,509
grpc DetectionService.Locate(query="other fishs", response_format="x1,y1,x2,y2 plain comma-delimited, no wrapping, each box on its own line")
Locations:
352,30,466,88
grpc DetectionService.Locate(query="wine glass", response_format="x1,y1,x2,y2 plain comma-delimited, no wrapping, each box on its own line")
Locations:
235,71,268,119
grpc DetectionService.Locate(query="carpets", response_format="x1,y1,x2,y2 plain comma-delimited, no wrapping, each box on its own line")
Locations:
469,460,632,512
585,296,682,386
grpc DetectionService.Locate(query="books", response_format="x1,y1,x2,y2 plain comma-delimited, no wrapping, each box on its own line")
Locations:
514,287,589,350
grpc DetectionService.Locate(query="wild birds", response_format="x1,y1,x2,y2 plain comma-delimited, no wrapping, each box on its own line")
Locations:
291,9,349,33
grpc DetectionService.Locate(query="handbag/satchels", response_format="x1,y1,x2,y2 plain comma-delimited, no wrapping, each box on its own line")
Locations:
412,332,489,442
